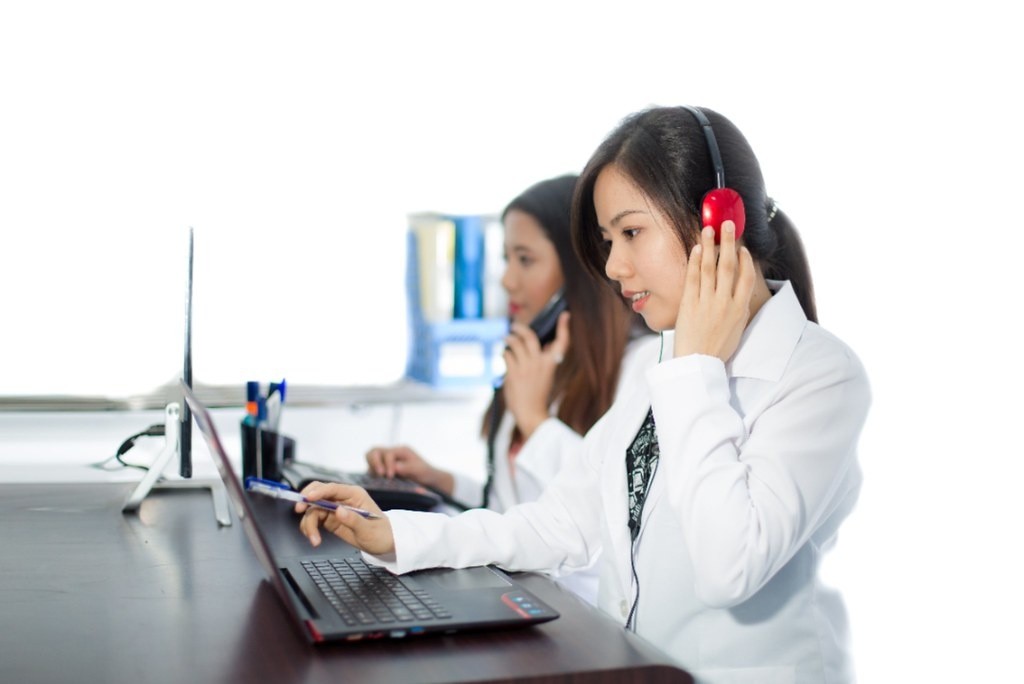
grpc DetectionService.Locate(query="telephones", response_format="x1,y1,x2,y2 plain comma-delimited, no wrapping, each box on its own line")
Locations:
494,278,580,359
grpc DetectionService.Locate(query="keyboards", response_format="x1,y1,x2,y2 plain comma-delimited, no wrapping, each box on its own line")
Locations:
280,460,439,512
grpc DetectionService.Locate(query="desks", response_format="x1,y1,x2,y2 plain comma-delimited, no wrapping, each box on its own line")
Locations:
0,474,700,684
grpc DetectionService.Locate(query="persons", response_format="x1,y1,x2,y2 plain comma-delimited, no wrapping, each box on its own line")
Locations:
369,175,663,606
296,106,872,684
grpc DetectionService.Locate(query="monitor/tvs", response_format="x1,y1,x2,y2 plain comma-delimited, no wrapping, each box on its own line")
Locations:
120,228,232,527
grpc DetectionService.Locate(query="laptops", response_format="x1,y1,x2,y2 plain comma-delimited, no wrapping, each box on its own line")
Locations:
179,379,562,645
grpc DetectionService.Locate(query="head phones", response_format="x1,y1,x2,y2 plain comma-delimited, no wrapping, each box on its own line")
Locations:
679,105,746,245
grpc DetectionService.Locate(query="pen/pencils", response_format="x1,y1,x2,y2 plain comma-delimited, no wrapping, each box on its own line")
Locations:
239,377,293,429
247,476,383,525
